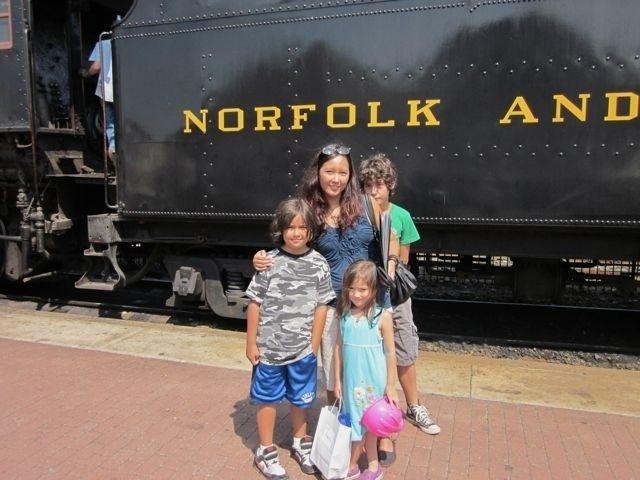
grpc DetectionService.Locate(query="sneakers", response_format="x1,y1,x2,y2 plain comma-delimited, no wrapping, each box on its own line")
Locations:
360,462,384,479
253,444,289,480
346,465,361,480
291,435,319,475
405,400,441,436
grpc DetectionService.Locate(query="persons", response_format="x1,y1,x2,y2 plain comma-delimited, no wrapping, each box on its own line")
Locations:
80,20,118,184
330,260,397,480
251,145,400,467
244,198,337,480
358,153,441,434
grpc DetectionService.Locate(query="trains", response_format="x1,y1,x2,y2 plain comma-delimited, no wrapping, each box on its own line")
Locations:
0,0,640,327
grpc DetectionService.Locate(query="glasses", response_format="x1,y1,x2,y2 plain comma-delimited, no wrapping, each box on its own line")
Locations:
321,145,352,155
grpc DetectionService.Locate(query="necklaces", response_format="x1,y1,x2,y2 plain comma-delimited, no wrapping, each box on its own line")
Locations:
326,207,340,226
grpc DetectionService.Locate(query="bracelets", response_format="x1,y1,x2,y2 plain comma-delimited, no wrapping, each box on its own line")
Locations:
389,258,397,265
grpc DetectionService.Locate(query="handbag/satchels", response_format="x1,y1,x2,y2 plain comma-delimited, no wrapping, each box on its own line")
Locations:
377,261,418,307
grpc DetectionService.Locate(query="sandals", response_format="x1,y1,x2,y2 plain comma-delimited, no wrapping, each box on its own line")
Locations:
378,437,396,467
